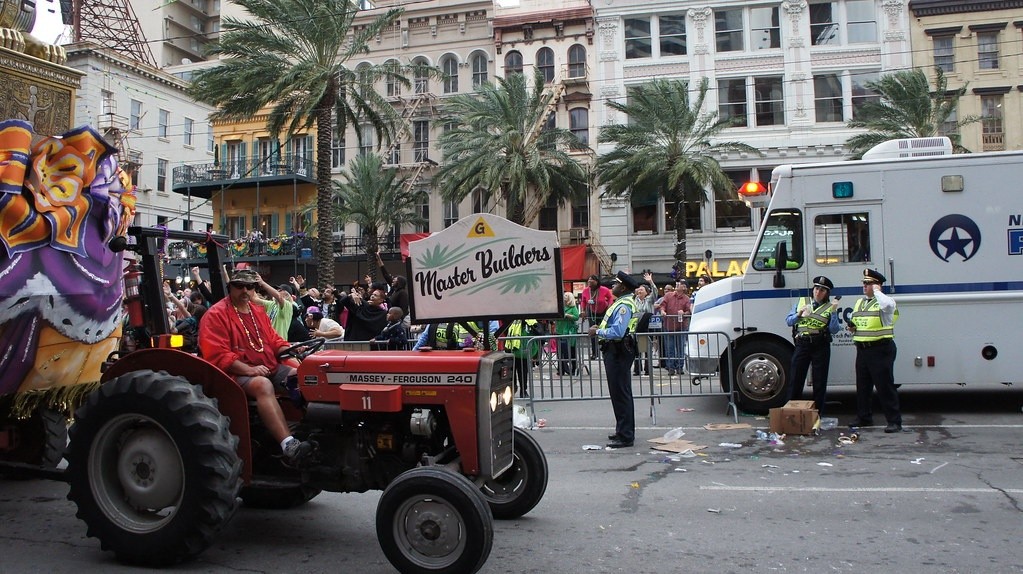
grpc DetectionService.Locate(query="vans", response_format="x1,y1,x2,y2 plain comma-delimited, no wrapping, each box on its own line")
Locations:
682,134,1023,417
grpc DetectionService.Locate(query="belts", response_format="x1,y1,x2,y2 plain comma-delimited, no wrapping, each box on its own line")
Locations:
798,333,823,343
855,338,891,348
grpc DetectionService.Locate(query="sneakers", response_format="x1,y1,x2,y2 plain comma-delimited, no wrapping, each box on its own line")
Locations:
885,423,902,432
848,418,874,426
283,438,311,463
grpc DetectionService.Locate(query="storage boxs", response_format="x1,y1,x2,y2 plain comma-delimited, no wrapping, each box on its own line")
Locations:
301,247,311,257
768,401,819,436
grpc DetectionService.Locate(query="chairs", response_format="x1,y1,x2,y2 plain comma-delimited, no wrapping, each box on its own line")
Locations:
191,169,204,182
538,344,559,371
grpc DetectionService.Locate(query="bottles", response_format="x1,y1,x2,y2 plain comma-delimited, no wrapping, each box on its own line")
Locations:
756,430,768,438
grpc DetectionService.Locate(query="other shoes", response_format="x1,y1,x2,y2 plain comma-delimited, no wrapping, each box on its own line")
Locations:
668,370,675,375
677,368,684,374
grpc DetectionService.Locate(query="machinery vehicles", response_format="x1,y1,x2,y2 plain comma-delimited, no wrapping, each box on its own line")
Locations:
62,224,552,574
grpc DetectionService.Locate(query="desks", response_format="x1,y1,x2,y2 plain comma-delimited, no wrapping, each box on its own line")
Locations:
271,164,292,175
207,170,227,180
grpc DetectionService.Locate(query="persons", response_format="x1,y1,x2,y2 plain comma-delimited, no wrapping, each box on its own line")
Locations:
197,263,314,461
222,246,410,352
764,249,800,268
846,266,902,433
783,276,839,416
632,273,657,376
163,266,215,354
554,291,580,375
409,315,543,399
580,276,614,360
587,271,640,447
654,264,714,375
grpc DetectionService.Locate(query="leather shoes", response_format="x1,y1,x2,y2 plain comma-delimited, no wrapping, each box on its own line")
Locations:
606,439,633,448
609,432,618,439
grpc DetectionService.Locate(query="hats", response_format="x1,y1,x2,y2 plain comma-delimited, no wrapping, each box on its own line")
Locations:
677,279,689,288
276,284,292,296
304,306,321,317
612,271,639,291
226,269,259,288
862,268,886,285
813,276,833,291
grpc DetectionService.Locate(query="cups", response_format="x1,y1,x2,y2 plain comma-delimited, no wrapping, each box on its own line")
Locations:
678,311,682,322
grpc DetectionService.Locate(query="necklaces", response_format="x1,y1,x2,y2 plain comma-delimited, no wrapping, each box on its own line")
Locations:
589,289,597,322
234,302,263,352
270,305,277,323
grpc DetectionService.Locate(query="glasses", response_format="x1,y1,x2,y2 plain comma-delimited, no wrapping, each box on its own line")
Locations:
863,280,874,285
230,282,256,290
277,289,284,292
